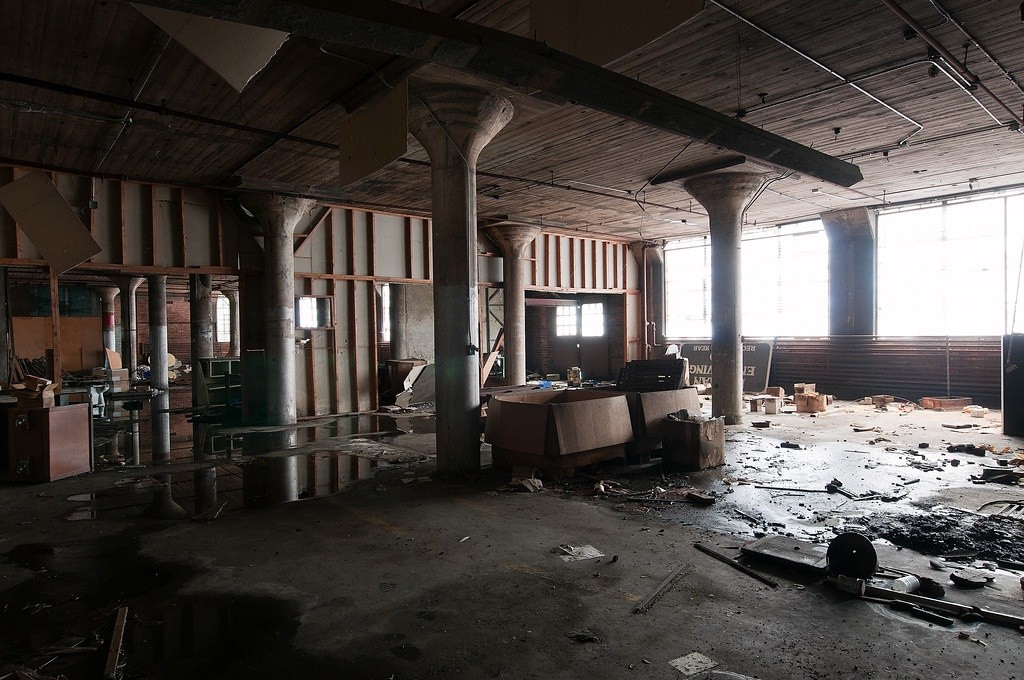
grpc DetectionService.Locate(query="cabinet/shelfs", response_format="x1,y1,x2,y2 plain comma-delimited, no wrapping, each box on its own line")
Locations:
6,402,96,485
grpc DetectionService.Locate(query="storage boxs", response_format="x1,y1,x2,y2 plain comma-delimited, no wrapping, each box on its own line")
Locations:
485,386,726,479
105,368,130,418
872,395,895,405
793,382,826,412
11,383,59,409
766,387,784,407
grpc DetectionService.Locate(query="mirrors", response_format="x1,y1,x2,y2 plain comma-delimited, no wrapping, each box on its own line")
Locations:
294,295,335,332
